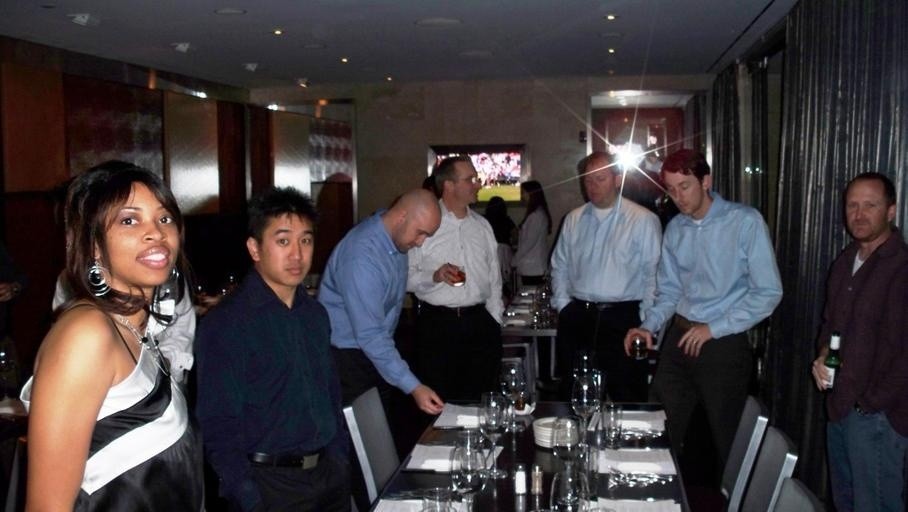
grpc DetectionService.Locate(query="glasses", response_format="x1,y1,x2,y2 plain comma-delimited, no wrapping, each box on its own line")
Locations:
453,175,479,186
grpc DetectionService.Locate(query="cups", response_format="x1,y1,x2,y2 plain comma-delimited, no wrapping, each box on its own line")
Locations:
450,266,467,289
630,334,650,363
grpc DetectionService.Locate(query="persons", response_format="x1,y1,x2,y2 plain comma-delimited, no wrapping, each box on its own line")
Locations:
812,173,908,512
20,150,785,512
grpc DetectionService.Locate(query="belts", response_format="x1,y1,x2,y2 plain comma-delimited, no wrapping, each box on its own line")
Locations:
670,313,709,336
247,451,322,472
411,293,486,317
566,294,643,315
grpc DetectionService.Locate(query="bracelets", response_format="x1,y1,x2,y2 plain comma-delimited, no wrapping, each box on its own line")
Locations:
854,402,866,416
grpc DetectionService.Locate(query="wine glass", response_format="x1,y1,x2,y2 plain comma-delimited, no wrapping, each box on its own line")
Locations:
421,365,526,511
550,356,622,512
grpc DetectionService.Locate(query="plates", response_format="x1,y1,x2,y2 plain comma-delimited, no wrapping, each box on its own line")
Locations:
533,416,576,447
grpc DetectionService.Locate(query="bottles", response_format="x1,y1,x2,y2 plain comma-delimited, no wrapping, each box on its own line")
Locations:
823,332,841,394
512,463,548,497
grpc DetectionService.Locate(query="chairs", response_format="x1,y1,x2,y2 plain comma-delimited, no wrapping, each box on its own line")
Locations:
342,385,401,505
773,477,827,512
740,425,799,512
498,337,539,397
718,393,770,512
